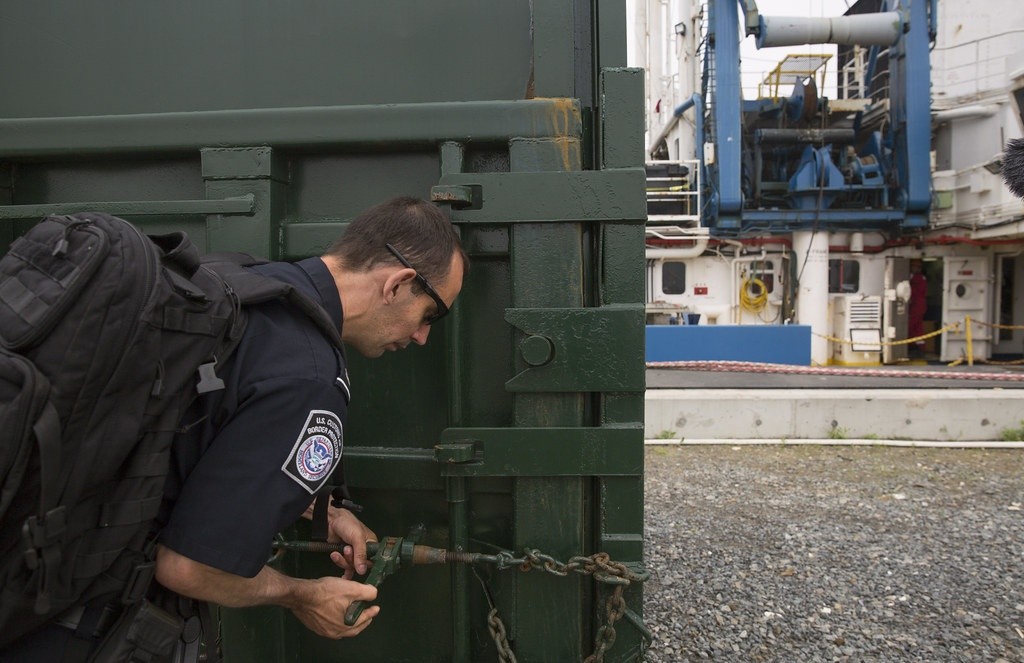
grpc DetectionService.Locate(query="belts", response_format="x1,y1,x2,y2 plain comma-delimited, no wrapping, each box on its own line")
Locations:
145,579,199,620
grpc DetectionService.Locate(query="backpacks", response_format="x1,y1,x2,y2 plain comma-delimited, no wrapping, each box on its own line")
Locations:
0,211,345,663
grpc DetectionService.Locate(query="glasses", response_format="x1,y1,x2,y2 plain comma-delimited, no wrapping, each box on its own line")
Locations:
387,243,449,326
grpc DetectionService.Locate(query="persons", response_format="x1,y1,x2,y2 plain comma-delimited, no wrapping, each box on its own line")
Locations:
910,258,928,359
0,195,470,662
